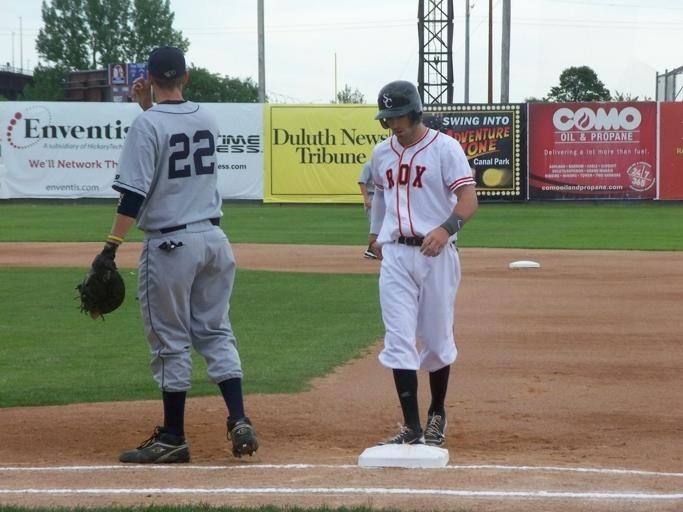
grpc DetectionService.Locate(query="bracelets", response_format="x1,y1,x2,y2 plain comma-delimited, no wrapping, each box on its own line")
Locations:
439,212,466,237
368,238,376,249
107,235,125,245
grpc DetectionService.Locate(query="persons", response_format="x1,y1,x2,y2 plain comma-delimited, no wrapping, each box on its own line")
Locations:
76,44,260,465
357,158,380,260
368,79,478,444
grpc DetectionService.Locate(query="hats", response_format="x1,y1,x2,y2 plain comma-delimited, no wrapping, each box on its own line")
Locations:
147,46,186,83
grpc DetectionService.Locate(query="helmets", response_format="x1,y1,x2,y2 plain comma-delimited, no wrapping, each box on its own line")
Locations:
373,80,422,129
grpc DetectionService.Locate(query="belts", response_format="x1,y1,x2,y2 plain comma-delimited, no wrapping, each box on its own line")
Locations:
392,236,423,246
160,217,220,234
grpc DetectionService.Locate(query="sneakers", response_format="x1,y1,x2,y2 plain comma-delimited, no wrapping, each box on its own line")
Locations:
377,427,425,447
364,249,378,260
424,413,448,443
118,428,190,463
225,418,258,458
